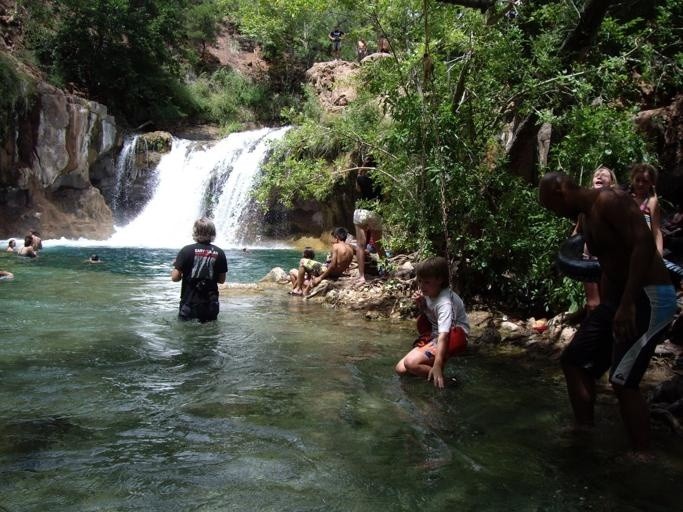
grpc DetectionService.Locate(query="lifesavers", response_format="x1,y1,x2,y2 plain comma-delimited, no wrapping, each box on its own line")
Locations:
556,233,682,283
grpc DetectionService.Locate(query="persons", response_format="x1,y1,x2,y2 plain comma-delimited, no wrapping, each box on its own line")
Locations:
353,156,389,282
7,229,42,258
89,254,99,263
260,227,378,294
395,256,470,388
172,217,228,322
0,270,11,276
